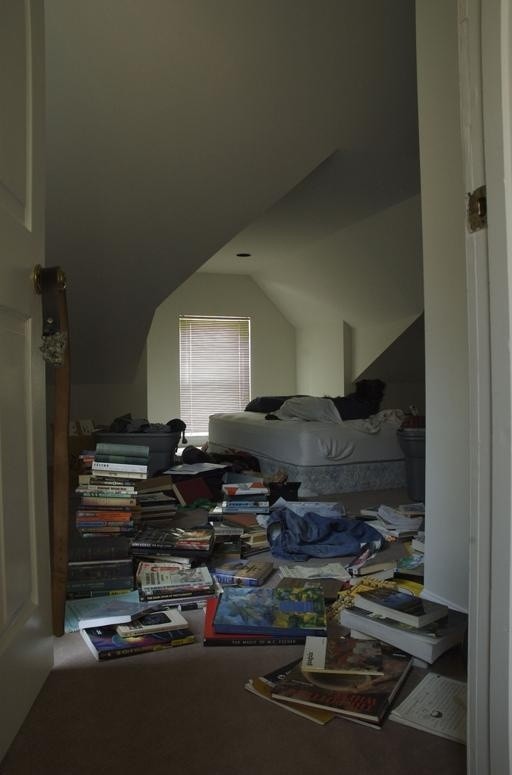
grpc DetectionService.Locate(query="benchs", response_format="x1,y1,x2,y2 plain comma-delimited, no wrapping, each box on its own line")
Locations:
53,418,110,456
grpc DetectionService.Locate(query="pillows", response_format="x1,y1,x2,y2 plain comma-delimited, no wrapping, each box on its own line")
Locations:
355,378,389,414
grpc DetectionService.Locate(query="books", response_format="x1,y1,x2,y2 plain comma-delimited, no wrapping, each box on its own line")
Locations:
58,418,469,741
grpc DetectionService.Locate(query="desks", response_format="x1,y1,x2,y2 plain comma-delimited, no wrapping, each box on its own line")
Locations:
394,428,424,502
92,428,182,477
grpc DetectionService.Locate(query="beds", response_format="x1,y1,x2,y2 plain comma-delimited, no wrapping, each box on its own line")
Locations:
206,394,415,498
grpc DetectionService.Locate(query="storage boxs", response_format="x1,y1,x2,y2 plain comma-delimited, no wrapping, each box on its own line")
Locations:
178,471,218,507
